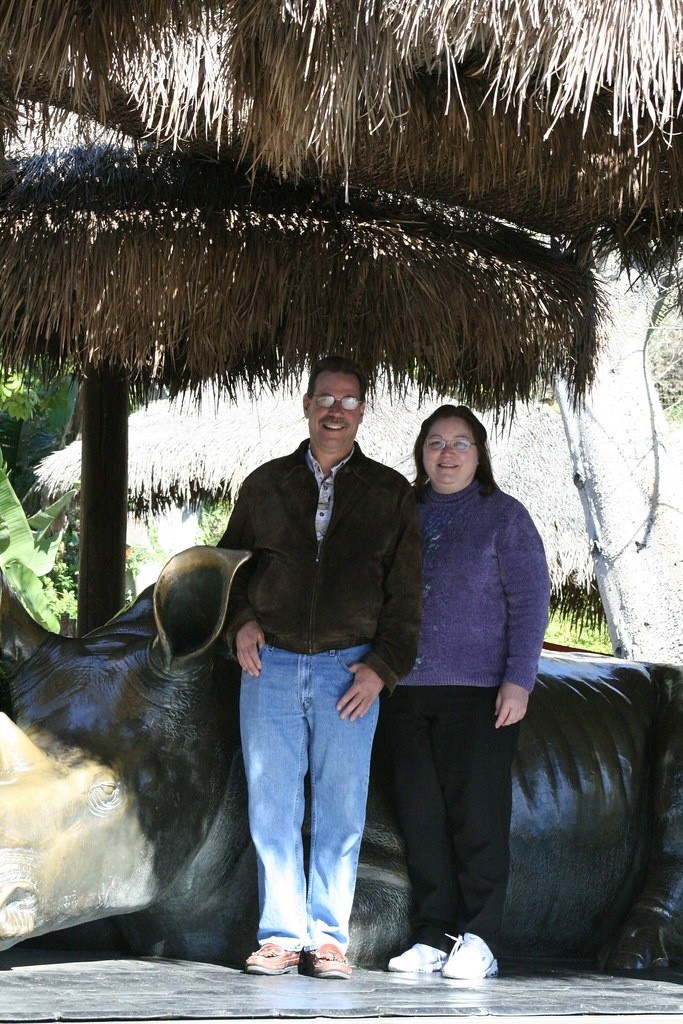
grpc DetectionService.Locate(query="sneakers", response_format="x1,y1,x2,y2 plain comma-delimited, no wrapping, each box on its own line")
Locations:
442,932,499,979
388,944,444,973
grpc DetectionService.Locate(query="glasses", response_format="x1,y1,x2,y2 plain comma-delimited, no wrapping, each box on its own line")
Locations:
311,395,370,407
426,435,482,448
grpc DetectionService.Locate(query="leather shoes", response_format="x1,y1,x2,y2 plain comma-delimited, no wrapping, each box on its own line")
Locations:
308,943,353,980
245,937,304,974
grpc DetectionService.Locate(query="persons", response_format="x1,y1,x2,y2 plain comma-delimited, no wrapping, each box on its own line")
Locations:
217,352,425,982
388,403,549,979
